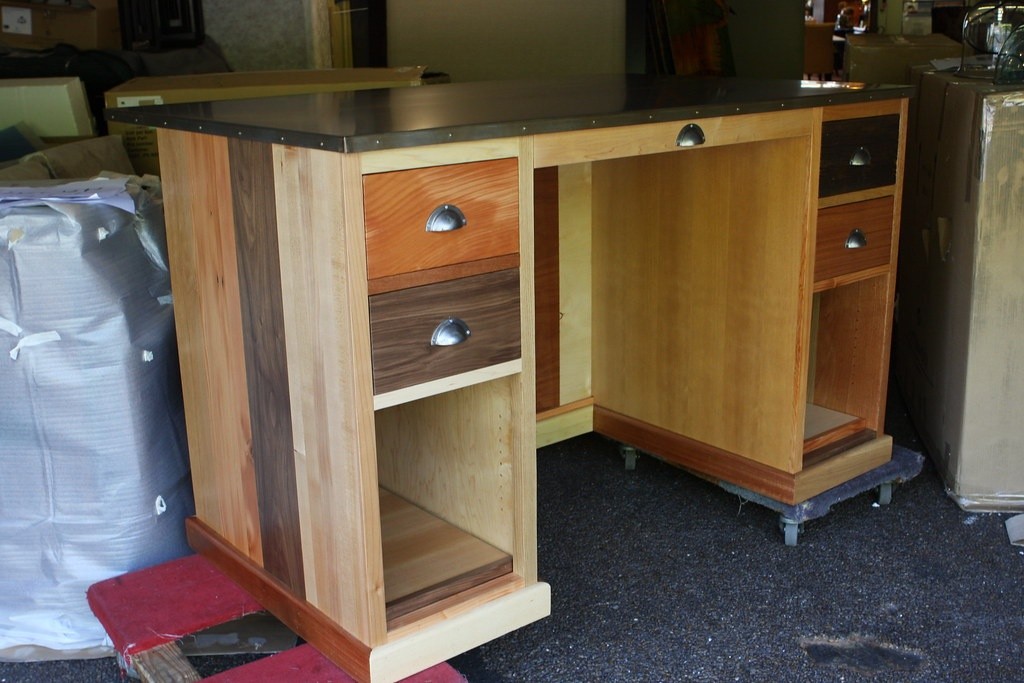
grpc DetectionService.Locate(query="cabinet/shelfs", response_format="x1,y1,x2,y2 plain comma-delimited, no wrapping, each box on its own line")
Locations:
103,75,913,683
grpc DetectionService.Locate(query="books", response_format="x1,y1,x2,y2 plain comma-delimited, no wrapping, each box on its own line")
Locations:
930,58,961,72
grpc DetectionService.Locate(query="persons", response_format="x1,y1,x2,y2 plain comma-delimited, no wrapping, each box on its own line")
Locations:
834,2,867,76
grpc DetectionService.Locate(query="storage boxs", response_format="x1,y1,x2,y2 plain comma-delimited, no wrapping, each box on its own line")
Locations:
907,56,1024,514
0,0,96,50
106,63,428,180
844,33,962,83
